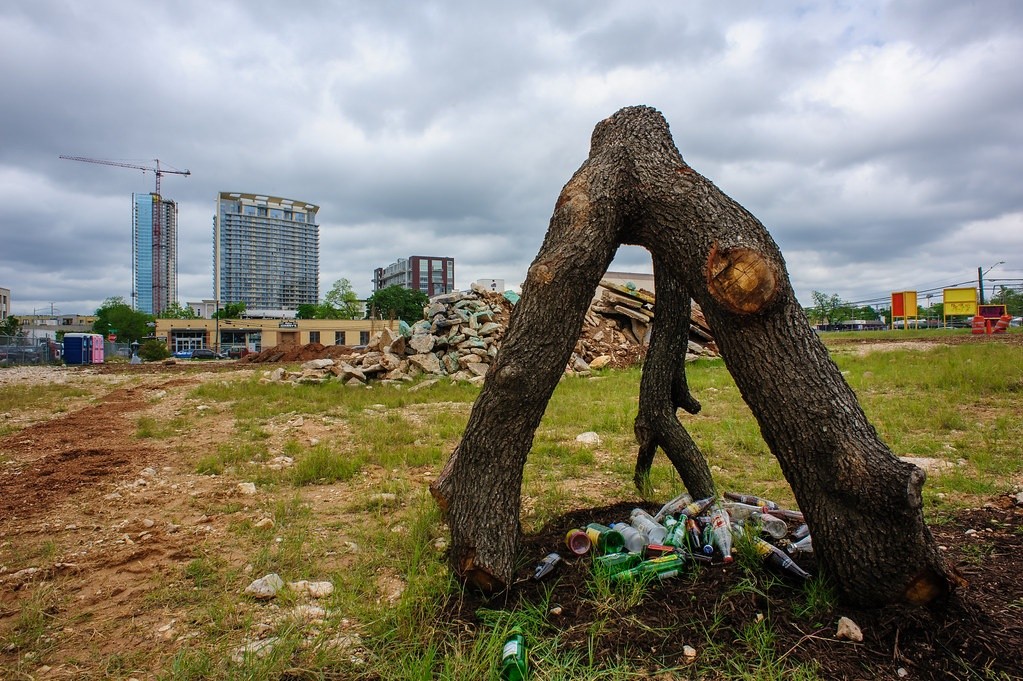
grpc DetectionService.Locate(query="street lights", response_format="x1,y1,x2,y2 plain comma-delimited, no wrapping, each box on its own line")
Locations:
215,300,219,353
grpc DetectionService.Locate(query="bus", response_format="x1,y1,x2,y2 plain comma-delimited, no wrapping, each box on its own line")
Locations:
41,343,64,355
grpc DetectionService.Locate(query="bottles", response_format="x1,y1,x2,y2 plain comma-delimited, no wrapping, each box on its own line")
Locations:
565,492,814,582
502,623,528,681
531,551,562,580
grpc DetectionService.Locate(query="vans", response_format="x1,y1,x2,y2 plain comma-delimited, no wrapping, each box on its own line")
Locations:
18,351,40,364
892,323,898,330
230,347,250,359
119,348,132,355
946,322,972,328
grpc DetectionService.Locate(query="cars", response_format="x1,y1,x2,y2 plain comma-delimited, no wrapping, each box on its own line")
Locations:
22,347,34,352
191,350,225,360
908,323,927,329
0,353,17,363
175,349,194,358
